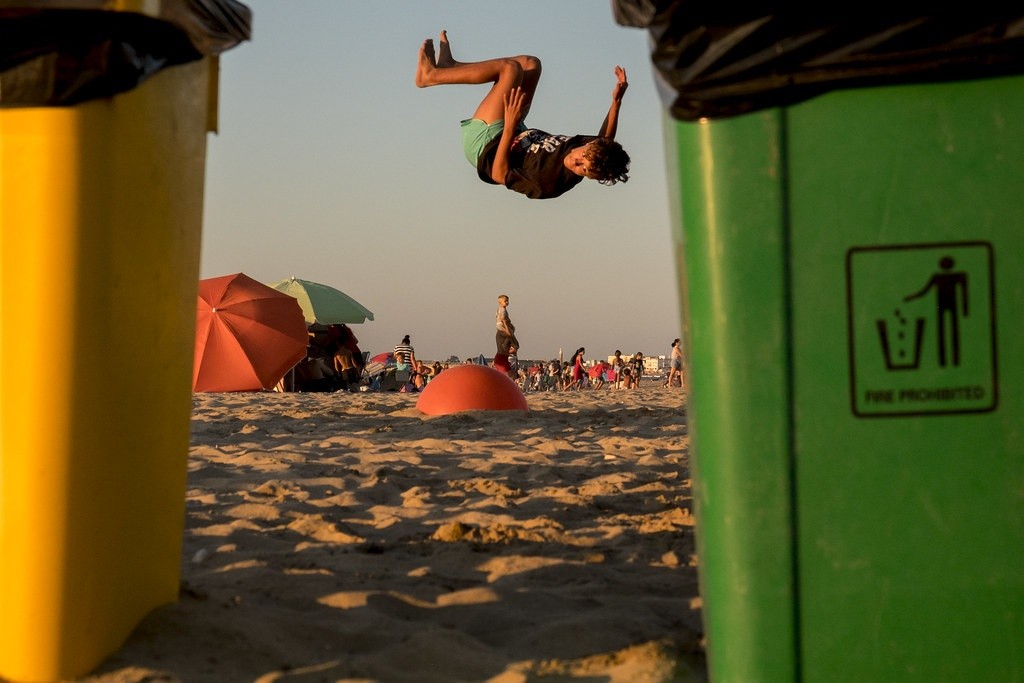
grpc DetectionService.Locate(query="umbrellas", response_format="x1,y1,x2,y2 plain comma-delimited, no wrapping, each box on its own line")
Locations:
265,276,374,325
191,271,309,393
361,352,393,378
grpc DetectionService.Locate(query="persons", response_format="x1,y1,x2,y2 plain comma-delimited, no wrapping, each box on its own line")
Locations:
493,295,519,378
415,30,630,199
668,339,685,388
393,335,448,388
333,338,360,386
465,347,645,392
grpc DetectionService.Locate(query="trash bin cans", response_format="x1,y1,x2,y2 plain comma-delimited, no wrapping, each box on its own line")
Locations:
613,0,1024,683
2,0,254,683
